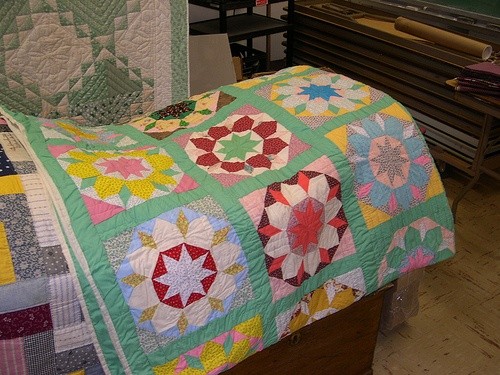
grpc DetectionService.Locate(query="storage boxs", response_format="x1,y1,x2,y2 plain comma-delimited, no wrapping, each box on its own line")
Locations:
229,42,267,80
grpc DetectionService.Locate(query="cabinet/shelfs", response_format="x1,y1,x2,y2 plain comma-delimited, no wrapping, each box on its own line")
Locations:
188,0,293,82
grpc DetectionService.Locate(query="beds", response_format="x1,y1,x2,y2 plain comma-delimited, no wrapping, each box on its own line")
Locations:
0,64,457,375
